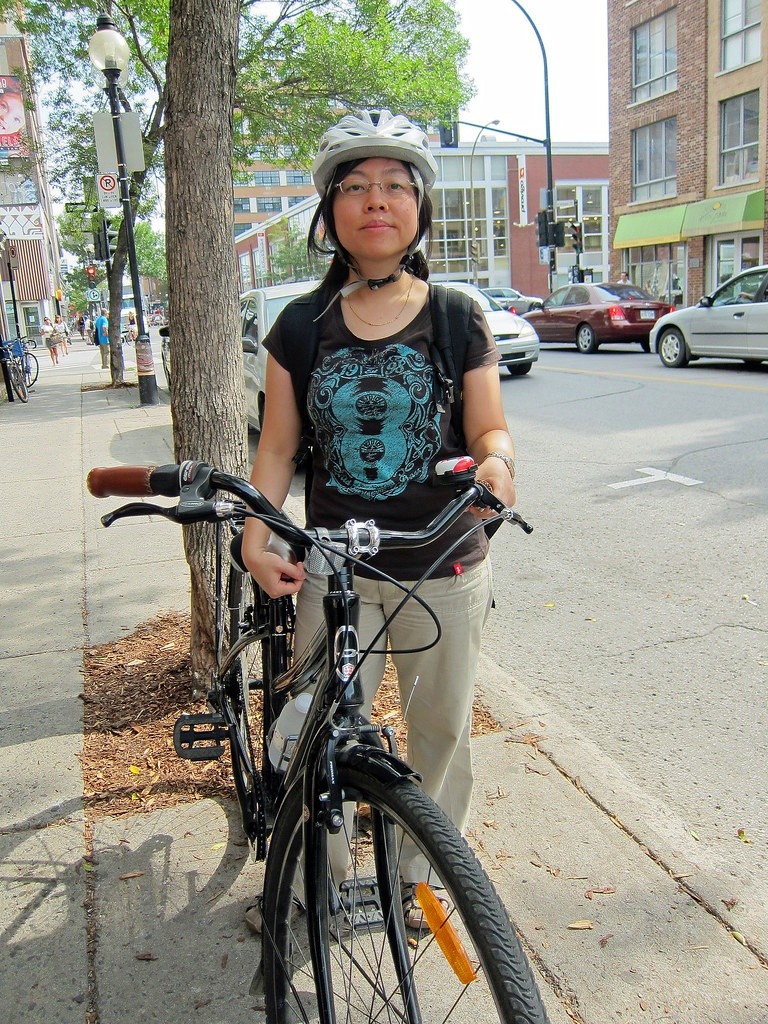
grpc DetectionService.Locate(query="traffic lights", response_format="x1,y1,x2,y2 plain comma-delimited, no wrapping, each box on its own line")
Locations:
571,224,583,252
88,266,96,290
93,217,117,262
555,221,566,246
533,210,547,247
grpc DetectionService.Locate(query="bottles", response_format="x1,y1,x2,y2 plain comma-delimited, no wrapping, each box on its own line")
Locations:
269,693,313,771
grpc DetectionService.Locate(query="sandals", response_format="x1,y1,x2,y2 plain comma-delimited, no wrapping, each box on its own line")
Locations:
245,888,304,933
402,882,449,928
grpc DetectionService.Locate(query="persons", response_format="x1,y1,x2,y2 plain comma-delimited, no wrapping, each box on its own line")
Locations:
617,272,632,286
41,317,59,366
128,312,136,345
0,87,25,134
53,315,68,357
78,317,85,340
241,109,516,934
95,308,109,370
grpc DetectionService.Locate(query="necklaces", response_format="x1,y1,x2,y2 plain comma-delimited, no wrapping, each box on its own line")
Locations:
348,281,414,326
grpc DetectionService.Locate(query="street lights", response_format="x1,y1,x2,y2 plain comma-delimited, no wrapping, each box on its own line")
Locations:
88,8,160,401
470,122,504,279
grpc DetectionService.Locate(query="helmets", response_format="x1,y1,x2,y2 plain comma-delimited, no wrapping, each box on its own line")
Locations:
312,109,438,198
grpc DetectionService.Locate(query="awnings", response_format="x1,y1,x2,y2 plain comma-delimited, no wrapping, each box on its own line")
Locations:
613,204,688,250
682,188,765,238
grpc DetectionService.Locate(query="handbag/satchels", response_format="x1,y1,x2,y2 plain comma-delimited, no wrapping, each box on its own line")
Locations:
94,327,99,345
50,333,63,346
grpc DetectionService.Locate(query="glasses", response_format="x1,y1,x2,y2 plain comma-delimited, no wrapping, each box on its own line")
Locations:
335,180,417,195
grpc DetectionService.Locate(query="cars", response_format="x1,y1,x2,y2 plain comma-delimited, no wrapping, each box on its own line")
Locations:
148,303,169,326
520,282,676,356
240,282,323,433
483,288,545,312
160,324,174,391
428,282,540,376
649,265,768,368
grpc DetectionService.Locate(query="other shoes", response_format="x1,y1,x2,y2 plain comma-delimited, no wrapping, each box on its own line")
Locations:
102,365,109,369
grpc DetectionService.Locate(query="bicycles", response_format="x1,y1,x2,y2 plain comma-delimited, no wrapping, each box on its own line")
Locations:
0,335,40,402
126,323,133,346
86,460,549,1024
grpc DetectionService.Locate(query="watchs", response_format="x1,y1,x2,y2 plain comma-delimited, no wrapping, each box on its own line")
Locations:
478,451,516,481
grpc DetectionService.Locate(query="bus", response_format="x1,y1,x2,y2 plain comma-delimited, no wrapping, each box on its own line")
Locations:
118,294,149,333
